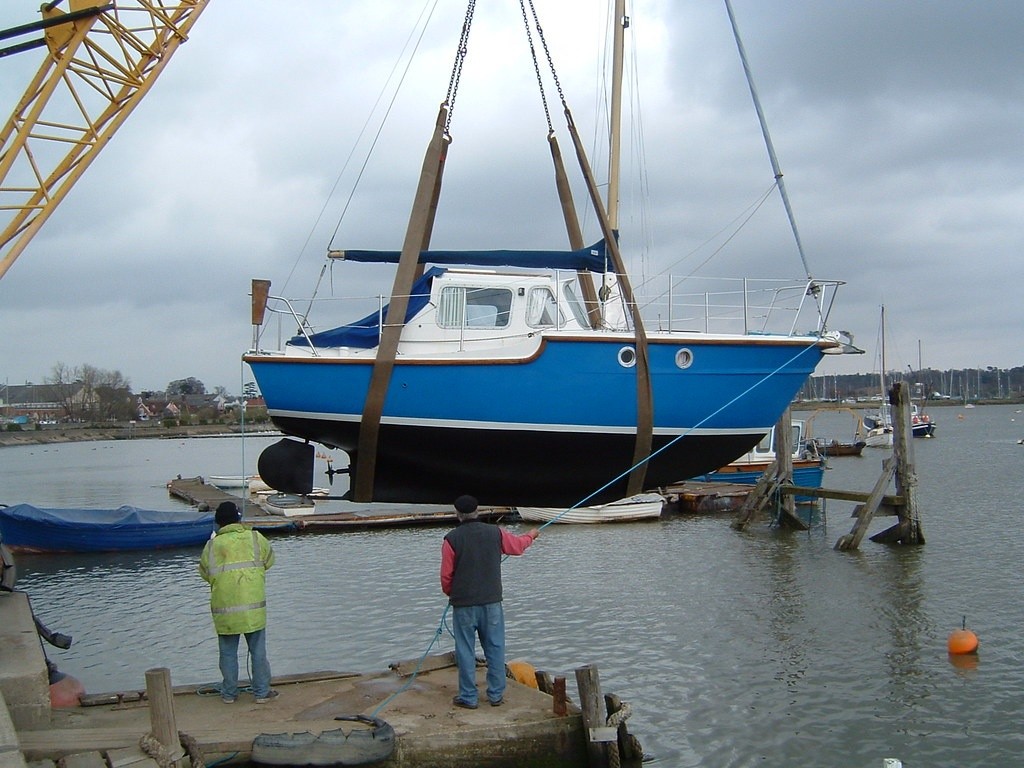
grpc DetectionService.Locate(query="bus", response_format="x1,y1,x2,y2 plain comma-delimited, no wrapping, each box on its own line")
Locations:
856,397,880,402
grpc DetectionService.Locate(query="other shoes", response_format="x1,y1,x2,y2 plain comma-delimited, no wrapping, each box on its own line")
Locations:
453,696,479,709
221,696,234,704
256,690,280,704
491,696,503,706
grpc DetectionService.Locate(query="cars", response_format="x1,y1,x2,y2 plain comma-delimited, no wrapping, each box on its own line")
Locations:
842,398,856,404
929,392,951,400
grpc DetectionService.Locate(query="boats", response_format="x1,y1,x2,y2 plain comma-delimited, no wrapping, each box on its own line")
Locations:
863,426,894,449
254,487,330,495
210,475,253,487
1013,410,1022,413
810,442,865,456
265,494,316,516
680,418,825,504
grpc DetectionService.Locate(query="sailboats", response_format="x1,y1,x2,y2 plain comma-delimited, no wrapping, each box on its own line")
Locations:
791,370,838,403
238,0,867,510
860,302,937,438
962,370,975,408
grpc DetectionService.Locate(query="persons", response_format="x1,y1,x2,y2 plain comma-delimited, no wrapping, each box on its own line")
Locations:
441,495,541,709
199,501,279,703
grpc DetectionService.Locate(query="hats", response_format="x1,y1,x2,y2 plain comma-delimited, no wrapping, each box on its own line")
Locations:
214,501,242,526
454,495,478,513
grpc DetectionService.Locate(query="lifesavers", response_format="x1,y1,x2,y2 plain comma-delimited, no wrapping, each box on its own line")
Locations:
923,417,928,423
913,417,917,423
